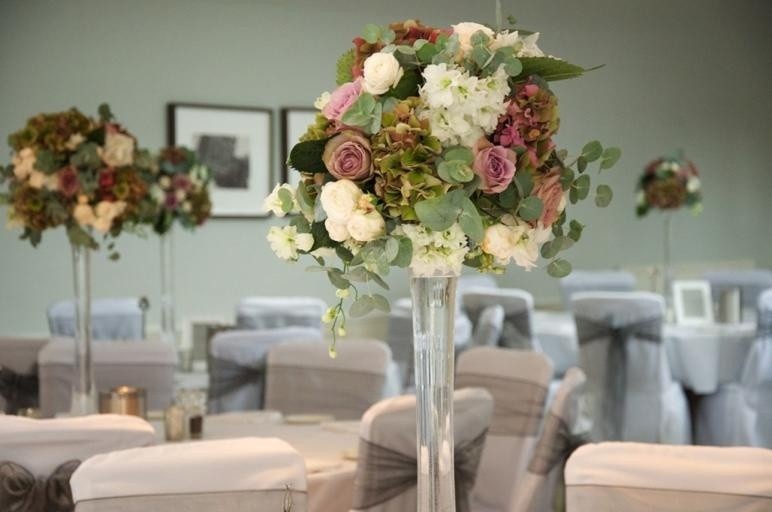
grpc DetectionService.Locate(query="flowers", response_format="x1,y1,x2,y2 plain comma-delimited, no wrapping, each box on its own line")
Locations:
632,148,706,215
0,107,219,240
264,22,611,360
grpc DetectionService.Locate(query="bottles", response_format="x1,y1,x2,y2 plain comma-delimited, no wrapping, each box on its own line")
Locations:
163,397,188,441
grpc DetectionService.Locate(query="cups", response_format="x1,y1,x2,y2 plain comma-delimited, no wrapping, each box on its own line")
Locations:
98,384,148,418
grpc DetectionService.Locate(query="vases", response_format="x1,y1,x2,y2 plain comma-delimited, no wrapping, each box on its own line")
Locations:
75,242,96,411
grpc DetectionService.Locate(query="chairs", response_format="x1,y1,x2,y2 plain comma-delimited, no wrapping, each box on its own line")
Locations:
563,441,772,512
458,288,539,349
560,273,771,444
0,335,179,416
205,298,412,418
46,299,149,343
347,387,494,512
451,348,586,512
0,414,310,512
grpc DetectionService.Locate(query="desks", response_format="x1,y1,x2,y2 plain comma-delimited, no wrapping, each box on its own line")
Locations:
150,410,363,488
175,371,209,391
532,313,756,347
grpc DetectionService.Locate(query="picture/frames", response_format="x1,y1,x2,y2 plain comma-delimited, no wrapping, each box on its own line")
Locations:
162,105,320,222
